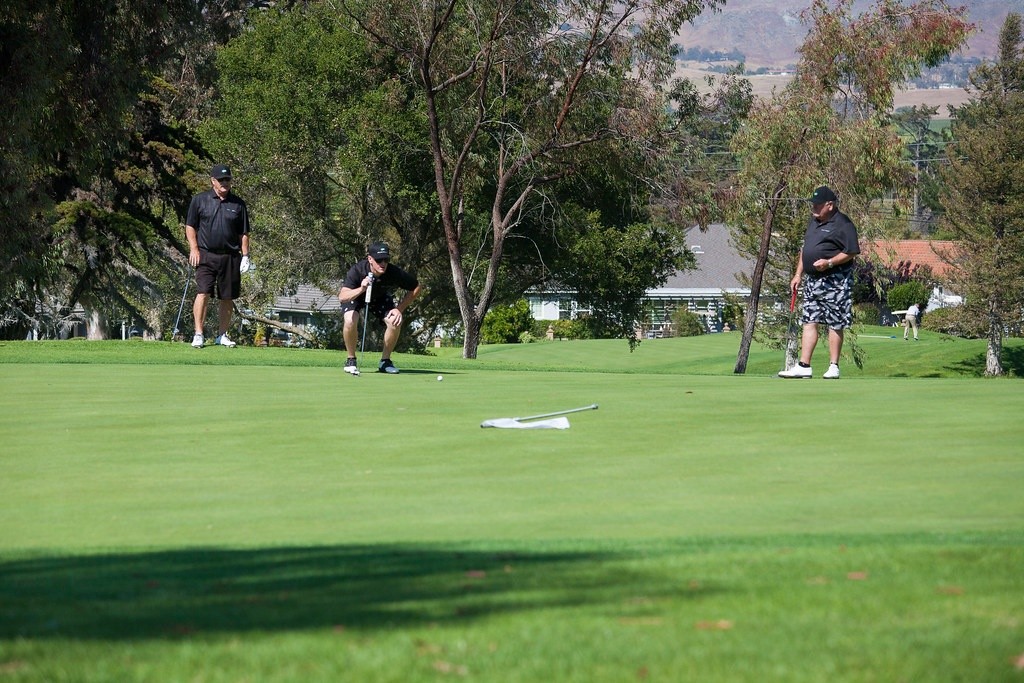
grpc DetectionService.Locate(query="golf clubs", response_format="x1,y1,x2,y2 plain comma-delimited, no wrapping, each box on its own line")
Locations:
772,292,797,378
171,268,195,343
350,272,373,376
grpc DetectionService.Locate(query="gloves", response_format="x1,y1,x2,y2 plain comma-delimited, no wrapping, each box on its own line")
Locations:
240,255,250,274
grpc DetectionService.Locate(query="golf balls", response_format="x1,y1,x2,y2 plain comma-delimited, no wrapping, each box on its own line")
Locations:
437,376,443,381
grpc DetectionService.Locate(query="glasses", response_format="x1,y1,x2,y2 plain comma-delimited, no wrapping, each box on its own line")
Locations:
217,178,230,182
375,258,390,263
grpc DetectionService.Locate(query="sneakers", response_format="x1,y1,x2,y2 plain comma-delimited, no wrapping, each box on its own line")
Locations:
378,360,399,374
344,358,360,375
191,332,204,349
778,363,812,379
823,365,840,379
214,332,237,348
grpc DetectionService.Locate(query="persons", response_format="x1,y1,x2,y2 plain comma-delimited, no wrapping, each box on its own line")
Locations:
904,304,920,340
186,165,252,348
778,186,860,379
338,241,421,373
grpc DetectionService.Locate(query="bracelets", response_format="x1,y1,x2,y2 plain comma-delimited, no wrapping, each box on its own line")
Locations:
795,274,800,276
828,259,834,268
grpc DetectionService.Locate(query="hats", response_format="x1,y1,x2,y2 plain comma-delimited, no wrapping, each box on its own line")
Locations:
805,186,837,204
210,165,233,179
368,242,390,259
